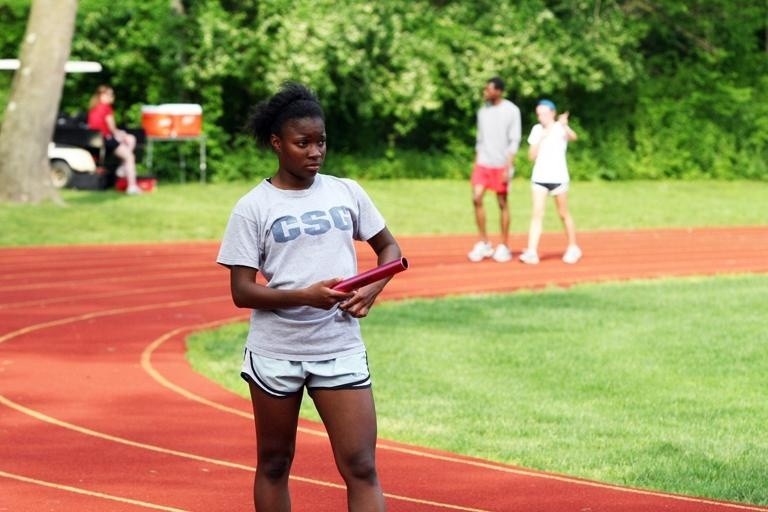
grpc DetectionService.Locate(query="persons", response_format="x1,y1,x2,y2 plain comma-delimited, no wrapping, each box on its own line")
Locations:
218,84,408,512
520,100,584,263
466,77,523,264
88,86,146,194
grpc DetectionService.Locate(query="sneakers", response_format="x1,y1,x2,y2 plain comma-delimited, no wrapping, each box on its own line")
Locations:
468,241,494,261
493,243,512,262
116,166,128,177
519,250,539,264
562,246,582,265
126,185,143,195
